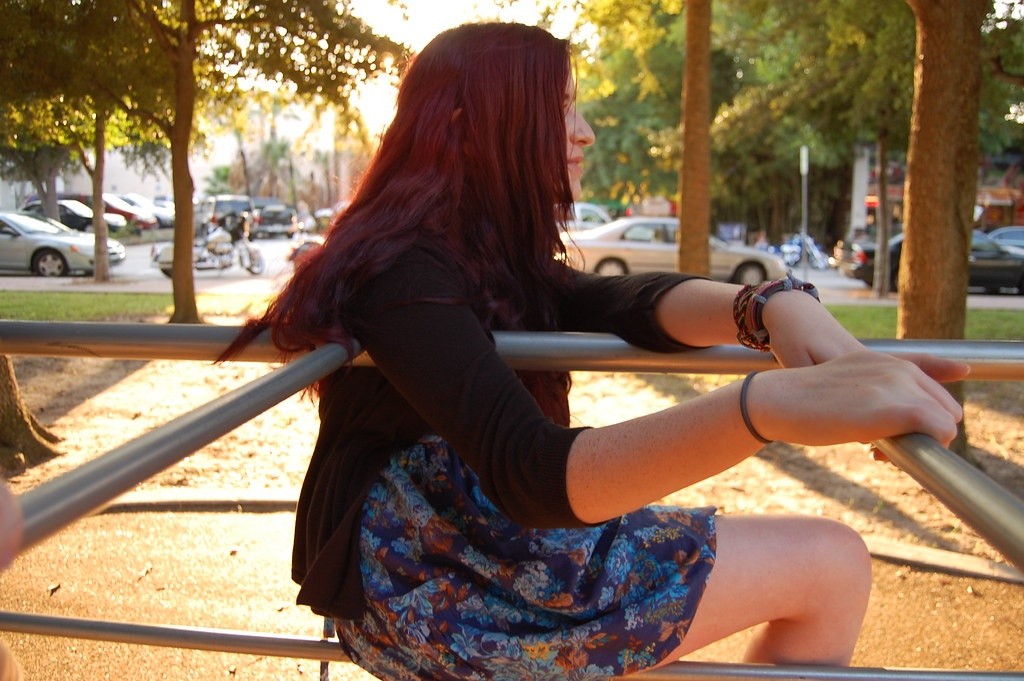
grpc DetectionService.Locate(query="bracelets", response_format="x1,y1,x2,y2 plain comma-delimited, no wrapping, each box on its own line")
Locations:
740,371,773,444
734,276,819,351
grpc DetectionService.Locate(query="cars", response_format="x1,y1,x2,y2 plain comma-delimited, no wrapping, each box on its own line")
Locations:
555,204,613,232
987,220,1024,250
121,188,199,228
828,226,1024,297
0,212,127,279
22,201,127,234
553,218,785,293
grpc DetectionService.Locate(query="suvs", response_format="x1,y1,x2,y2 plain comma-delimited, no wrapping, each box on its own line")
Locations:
202,193,255,238
27,192,155,234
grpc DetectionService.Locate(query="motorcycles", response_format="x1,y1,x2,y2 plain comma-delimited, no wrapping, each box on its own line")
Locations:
756,232,804,266
798,233,828,274
147,210,268,276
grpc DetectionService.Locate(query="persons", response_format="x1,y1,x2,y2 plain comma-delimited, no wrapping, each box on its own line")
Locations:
215,23,963,681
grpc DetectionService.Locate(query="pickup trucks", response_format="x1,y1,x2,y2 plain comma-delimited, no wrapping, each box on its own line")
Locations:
254,194,298,239
